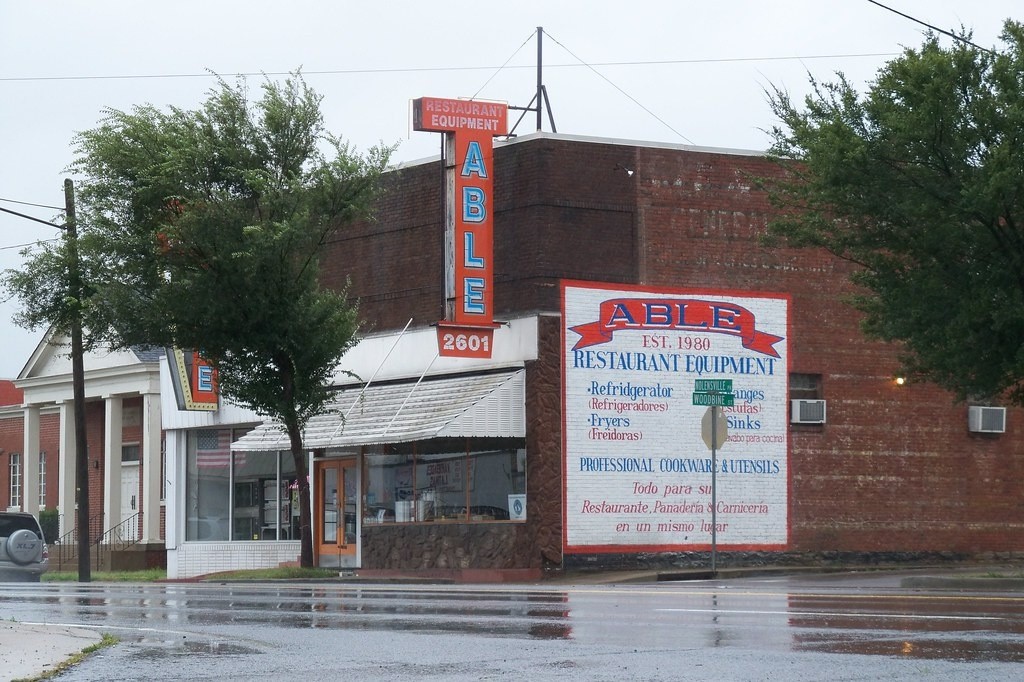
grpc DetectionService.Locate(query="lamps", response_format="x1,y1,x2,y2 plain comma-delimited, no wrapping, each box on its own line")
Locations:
613,163,634,177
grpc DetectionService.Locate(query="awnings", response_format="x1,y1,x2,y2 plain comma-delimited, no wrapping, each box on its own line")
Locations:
230,369,526,451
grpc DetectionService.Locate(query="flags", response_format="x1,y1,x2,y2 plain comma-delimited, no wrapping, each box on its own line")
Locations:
196,430,246,467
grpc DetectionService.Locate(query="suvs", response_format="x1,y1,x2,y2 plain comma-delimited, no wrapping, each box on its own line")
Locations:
0,509,49,584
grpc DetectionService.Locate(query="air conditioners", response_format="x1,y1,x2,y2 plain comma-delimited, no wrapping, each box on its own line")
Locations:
968,405,1006,434
789,399,826,424
